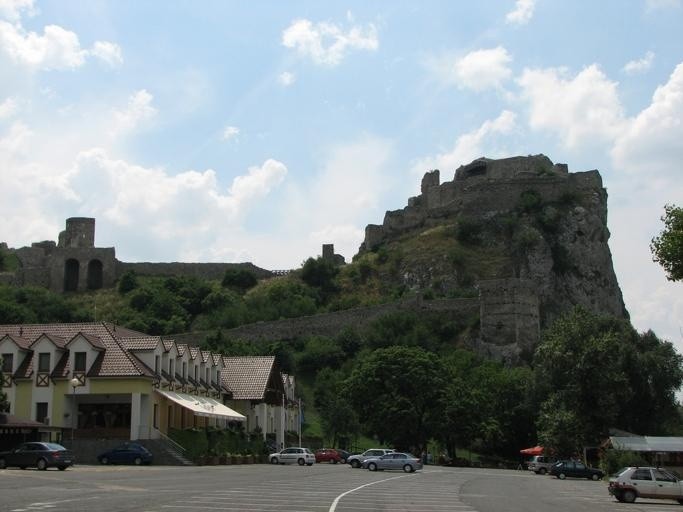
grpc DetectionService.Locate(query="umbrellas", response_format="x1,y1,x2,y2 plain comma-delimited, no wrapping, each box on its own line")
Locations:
520,447,544,454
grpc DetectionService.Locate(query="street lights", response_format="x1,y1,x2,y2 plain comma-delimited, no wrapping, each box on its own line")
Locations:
70,377,80,448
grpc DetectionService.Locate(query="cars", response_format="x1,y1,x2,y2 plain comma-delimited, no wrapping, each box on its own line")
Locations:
547,460,606,481
336,449,350,464
268,447,315,466
0,441,76,471
363,452,423,473
607,466,683,506
313,448,341,464
97,443,152,465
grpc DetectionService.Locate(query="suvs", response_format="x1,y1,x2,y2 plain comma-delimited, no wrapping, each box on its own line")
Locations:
347,448,399,468
527,455,554,475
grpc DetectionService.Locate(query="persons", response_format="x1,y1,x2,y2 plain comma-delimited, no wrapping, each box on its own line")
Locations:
422,451,426,463
427,451,433,465
